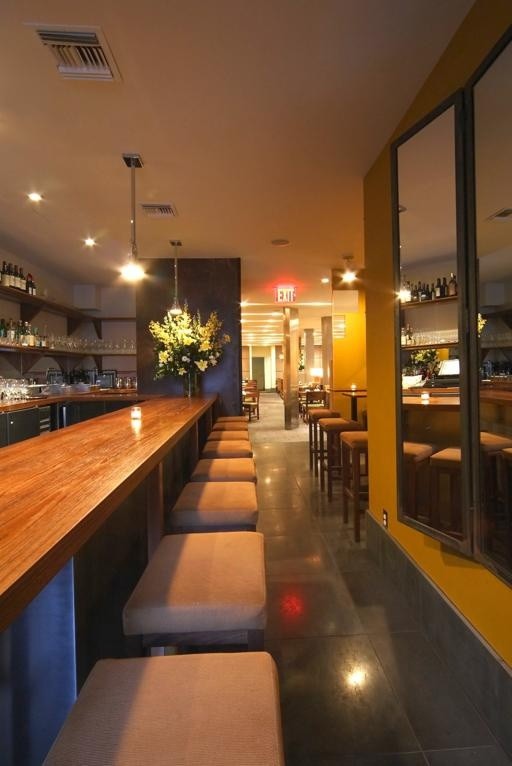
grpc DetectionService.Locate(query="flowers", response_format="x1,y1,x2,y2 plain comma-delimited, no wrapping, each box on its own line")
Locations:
412,349,441,376
148,300,231,379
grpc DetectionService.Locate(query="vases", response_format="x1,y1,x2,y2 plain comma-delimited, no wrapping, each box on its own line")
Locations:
181,371,198,397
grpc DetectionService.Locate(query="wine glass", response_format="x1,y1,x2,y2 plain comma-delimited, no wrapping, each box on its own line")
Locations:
0,377,27,402
47,332,136,355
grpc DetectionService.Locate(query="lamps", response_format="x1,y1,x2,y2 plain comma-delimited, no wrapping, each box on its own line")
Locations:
169,240,183,315
120,154,147,283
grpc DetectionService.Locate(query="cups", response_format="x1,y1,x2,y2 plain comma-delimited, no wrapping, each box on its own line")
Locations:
415,326,458,346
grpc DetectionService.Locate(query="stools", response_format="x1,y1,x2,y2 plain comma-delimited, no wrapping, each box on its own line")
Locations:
216,416,247,421
213,421,249,431
201,440,254,457
318,417,363,497
120,529,271,653
190,457,258,486
36,650,290,766
337,429,368,544
403,442,462,529
169,481,261,533
480,432,512,531
208,430,250,440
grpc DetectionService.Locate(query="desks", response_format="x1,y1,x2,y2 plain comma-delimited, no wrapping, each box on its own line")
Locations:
329,386,367,417
0,393,217,631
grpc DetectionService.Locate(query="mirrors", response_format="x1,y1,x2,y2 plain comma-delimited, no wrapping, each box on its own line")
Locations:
464,30,512,587
389,86,473,557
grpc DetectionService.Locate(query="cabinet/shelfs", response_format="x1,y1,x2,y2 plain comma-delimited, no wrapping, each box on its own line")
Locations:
0,287,136,410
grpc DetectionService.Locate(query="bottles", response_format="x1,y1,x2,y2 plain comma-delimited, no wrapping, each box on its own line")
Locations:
403,273,458,301
488,359,493,375
1,260,37,296
401,323,413,344
114,375,137,388
402,356,432,382
0,317,49,351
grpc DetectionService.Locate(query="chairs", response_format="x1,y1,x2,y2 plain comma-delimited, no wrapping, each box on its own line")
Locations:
298,382,327,423
242,378,261,422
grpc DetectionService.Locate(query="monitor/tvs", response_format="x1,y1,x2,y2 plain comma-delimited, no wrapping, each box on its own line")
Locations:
438,359,460,377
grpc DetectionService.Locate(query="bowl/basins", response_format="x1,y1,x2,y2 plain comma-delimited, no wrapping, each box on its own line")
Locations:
25,383,47,394
50,383,102,395
